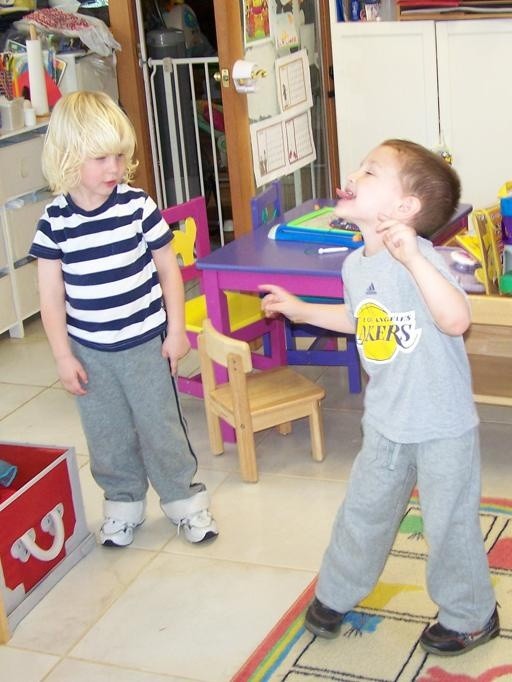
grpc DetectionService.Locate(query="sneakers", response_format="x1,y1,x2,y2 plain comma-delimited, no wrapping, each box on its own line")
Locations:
99,516,144,545
304,602,345,638
419,602,499,656
178,511,219,542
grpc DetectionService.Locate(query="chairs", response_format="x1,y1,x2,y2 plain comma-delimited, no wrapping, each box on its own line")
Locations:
250,178,362,395
196,317,327,485
158,197,288,443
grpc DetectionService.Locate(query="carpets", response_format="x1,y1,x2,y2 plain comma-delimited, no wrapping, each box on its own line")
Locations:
231,483,512,682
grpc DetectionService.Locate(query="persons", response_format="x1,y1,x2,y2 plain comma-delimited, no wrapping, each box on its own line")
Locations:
256,136,502,659
27,88,223,550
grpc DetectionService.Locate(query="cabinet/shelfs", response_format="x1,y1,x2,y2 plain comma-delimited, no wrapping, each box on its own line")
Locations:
0,113,57,339
396,0,512,20
328,0,512,236
463,284,511,409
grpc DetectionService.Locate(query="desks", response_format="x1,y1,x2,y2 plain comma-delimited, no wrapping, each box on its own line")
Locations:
194,197,473,445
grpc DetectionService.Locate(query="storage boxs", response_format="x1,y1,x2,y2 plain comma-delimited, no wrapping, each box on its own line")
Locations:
0,442,90,616
55,51,119,106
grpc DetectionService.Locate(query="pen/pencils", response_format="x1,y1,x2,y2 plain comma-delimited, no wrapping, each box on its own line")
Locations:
318,247,348,254
